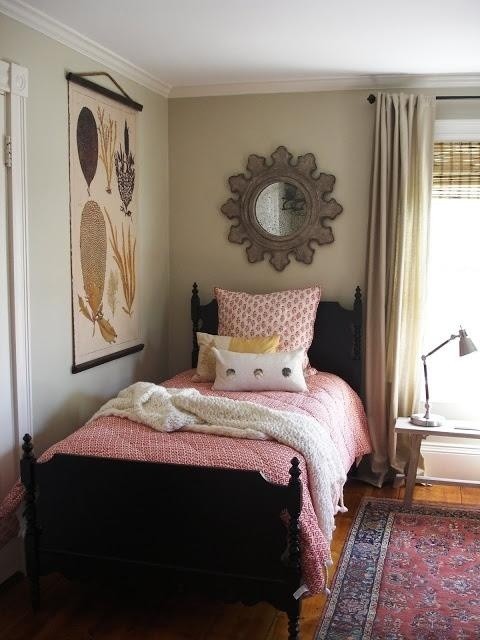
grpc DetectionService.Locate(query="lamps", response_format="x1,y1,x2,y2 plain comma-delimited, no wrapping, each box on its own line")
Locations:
409,325,478,427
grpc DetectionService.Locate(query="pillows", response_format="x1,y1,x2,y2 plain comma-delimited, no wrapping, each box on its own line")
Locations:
191,281,322,392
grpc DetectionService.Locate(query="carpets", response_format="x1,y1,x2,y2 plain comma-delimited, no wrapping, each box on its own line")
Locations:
312,495,480,640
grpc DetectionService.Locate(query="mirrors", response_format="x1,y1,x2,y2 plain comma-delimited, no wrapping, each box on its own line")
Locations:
220,146,343,270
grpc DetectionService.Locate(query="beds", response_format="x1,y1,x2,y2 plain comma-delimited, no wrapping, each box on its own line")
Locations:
1,283,375,640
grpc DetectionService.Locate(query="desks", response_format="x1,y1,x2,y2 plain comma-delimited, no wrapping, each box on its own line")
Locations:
394,416,480,500
